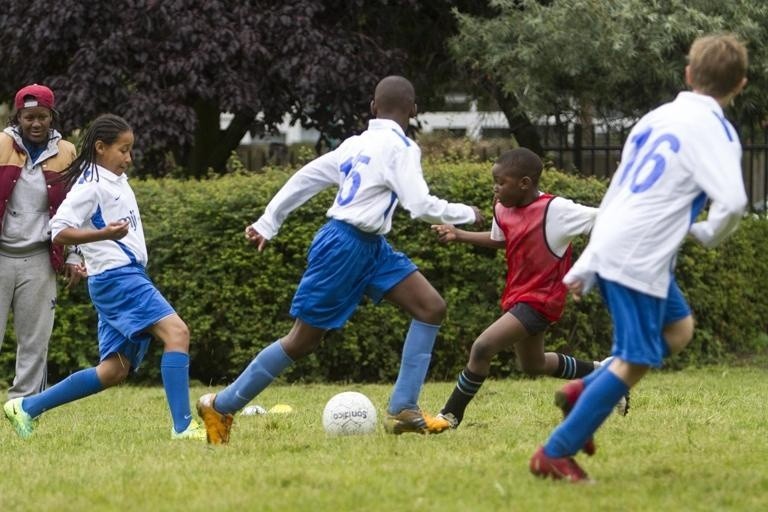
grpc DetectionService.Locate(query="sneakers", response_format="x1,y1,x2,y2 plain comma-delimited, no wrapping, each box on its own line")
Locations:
196,394,234,446
171,418,208,445
383,407,459,435
529,356,629,483
3,398,38,442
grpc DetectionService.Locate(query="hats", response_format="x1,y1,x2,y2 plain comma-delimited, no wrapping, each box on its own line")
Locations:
15,83,59,117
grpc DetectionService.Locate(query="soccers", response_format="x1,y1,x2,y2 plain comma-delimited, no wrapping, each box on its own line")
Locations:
322,390,377,436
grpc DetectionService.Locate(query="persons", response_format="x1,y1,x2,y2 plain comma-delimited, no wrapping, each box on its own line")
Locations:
192,73,482,448
0,81,80,399
430,144,631,431
1,111,207,443
527,31,751,484
